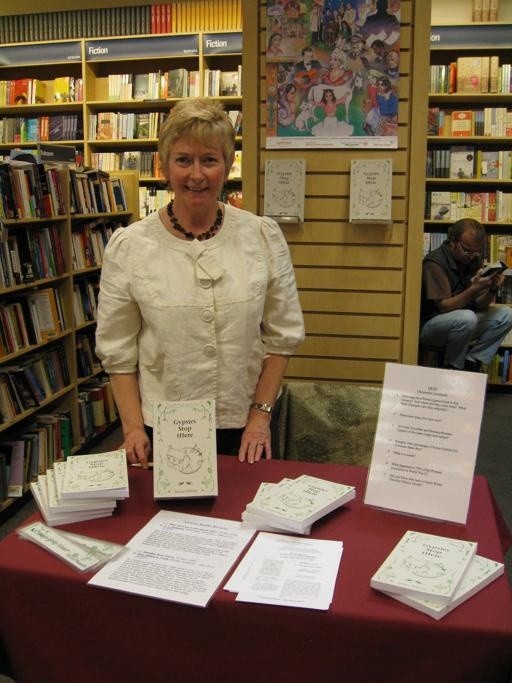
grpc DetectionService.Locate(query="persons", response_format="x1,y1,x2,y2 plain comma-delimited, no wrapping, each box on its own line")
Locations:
93,98,307,468
263,0,397,137
418,216,512,370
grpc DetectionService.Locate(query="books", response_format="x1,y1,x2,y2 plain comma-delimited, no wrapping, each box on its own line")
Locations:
470,259,507,281
31,449,129,527
1,223,124,499
348,158,390,225
1,68,239,216
425,56,510,386
0,1,243,44
240,474,355,536
369,530,506,620
153,401,218,499
263,158,306,225
473,1,500,22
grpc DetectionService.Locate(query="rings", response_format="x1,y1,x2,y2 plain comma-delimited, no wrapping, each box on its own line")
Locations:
256,440,267,447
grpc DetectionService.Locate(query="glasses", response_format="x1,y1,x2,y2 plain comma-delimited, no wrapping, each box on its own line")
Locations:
460,244,482,256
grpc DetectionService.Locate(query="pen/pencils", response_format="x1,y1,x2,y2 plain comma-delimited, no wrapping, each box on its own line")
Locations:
128,462,154,468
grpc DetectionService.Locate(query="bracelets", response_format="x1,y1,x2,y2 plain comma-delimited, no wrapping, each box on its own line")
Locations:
246,399,277,416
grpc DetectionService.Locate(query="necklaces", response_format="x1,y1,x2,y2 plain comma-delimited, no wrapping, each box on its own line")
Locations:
164,198,224,244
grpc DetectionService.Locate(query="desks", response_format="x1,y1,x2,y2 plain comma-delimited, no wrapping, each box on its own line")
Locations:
0,455,512,683
